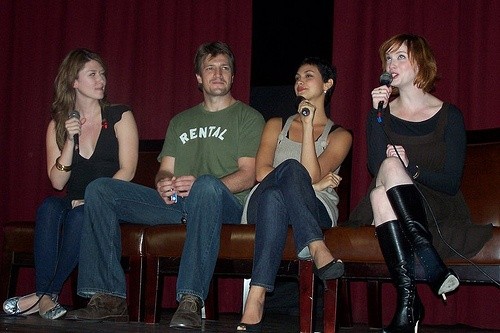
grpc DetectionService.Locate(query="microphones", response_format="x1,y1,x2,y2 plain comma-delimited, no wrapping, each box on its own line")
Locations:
68,111,80,154
377,73,393,123
296,96,310,117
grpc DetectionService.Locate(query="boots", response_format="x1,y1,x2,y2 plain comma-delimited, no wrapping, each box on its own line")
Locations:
375,218,425,333
386,182,461,304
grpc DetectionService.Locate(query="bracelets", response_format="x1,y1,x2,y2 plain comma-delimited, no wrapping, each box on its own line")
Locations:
56,157,73,173
413,165,421,180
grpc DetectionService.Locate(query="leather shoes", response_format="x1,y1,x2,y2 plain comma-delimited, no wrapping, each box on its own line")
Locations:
65,291,130,323
168,295,203,331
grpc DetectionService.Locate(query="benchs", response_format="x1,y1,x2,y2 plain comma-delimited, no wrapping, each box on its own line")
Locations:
147,152,348,333
0,143,159,323
322,141,500,333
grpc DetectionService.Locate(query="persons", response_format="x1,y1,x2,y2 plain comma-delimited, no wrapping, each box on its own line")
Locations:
236,55,353,333
68,42,265,329
350,33,493,333
4,48,139,320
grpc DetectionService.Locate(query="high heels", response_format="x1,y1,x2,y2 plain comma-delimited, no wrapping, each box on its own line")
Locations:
312,256,344,290
235,309,264,331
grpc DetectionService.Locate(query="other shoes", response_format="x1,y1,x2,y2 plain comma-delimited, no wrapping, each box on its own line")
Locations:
38,301,67,319
2,291,59,316
